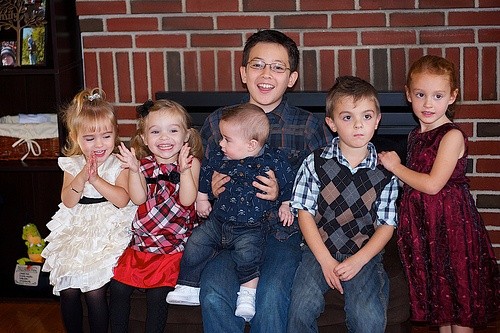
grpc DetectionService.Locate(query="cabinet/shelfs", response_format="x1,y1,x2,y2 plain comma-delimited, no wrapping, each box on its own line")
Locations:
0,0,84,299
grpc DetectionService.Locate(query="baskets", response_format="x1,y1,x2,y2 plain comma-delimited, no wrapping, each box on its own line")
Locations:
0,135,61,161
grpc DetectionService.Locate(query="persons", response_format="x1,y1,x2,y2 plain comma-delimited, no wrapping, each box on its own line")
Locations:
41,88,139,333
0,35,37,65
286,76,401,333
377,55,494,333
198,30,334,333
108,99,203,333
166,103,297,321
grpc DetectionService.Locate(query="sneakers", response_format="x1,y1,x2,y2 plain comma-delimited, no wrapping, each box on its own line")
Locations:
235,291,256,322
166,285,201,306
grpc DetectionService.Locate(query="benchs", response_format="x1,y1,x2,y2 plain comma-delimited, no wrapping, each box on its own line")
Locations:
107,91,421,333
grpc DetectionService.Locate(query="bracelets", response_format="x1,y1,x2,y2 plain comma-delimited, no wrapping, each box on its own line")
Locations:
70,184,83,193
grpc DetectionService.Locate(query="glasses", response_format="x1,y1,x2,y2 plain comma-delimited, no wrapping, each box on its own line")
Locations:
248,56,291,73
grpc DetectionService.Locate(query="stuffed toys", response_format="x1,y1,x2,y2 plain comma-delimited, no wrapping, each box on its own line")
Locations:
17,223,45,265
